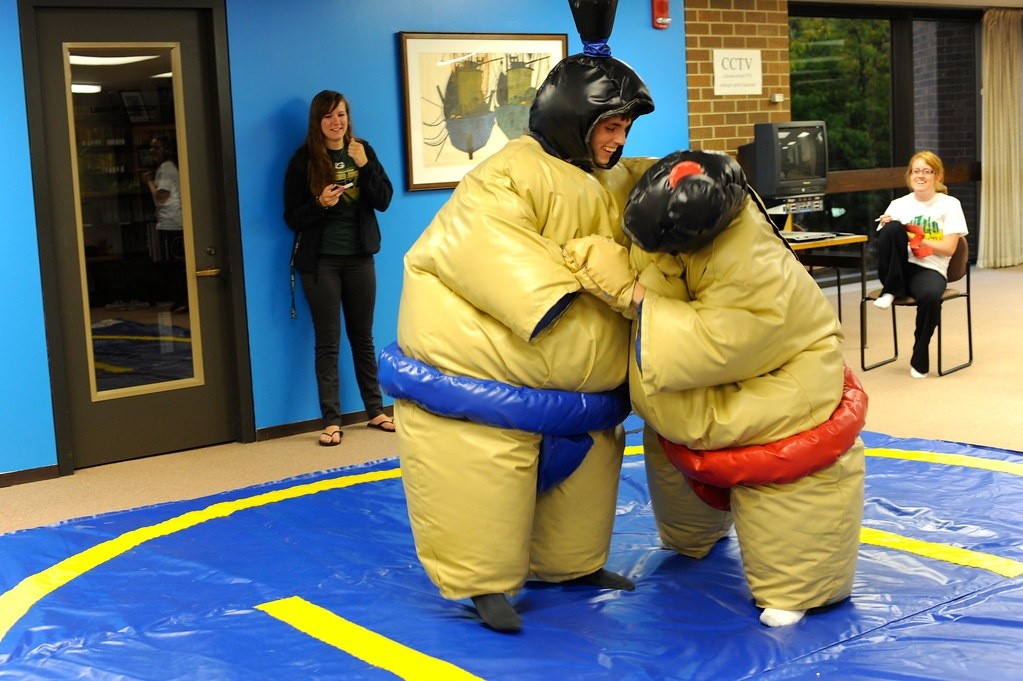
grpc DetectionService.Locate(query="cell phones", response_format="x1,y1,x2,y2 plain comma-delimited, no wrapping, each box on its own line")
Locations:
337,182,355,192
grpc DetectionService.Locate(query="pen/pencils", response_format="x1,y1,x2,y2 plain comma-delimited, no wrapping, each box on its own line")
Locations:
875,218,880,222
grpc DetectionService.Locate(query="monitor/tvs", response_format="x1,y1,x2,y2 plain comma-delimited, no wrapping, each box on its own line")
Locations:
738,121,828,197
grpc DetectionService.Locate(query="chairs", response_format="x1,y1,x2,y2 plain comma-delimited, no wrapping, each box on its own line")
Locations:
859,236,973,375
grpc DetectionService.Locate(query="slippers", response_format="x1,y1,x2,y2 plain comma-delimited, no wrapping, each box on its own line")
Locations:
320,429,343,447
366,418,395,432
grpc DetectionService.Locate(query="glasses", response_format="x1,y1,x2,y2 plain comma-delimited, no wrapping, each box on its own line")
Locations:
911,168,936,176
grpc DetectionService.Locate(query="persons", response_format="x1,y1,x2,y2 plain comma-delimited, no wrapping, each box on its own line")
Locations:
282,90,396,446
564,150,868,625
140,136,189,314
873,151,970,378
378,0,656,635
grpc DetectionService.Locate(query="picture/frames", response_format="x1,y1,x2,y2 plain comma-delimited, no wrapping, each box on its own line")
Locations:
120,90,150,122
396,31,569,192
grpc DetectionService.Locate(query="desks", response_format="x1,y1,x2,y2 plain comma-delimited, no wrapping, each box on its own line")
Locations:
784,230,869,348
